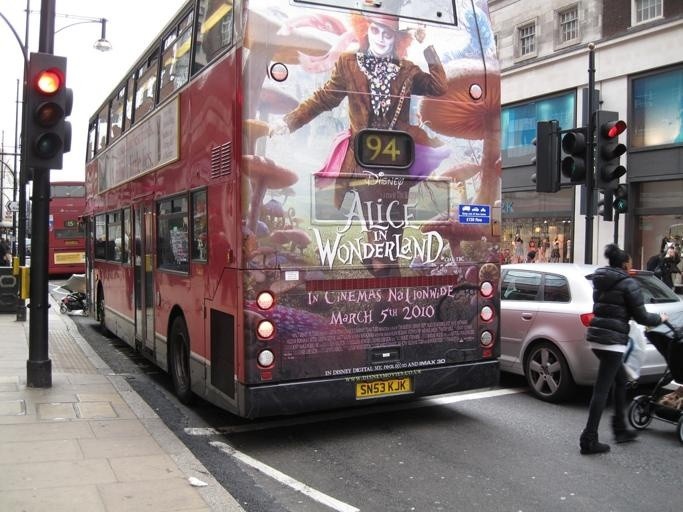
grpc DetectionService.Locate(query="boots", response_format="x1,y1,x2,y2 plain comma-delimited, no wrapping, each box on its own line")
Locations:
612,415,638,441
580,427,610,454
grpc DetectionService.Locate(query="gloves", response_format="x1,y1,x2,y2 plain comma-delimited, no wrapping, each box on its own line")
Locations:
267,114,290,139
413,28,434,53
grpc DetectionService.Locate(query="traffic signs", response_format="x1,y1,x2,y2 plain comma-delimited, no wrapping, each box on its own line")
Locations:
5,201,29,212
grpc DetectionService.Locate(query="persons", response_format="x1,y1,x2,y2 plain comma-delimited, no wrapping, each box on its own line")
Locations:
268,20,449,275
654,246,681,288
515,234,572,262
580,243,668,454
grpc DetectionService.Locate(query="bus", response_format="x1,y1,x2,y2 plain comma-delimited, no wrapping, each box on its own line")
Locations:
78,1,503,421
48,181,87,275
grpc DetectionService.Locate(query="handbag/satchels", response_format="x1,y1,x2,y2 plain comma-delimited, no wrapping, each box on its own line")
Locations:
622,336,644,381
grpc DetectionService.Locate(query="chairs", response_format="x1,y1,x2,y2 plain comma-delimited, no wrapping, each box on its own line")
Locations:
507,279,535,300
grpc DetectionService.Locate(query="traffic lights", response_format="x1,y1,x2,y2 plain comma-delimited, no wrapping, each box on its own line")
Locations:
613,182,629,213
26,52,67,170
530,110,627,193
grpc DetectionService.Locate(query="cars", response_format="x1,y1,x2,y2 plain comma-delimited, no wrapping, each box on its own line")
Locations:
500,262,683,403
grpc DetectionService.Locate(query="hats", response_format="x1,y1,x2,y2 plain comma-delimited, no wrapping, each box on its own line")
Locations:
365,12,399,31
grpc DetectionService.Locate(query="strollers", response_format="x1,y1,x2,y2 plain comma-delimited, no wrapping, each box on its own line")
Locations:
627,320,683,446
